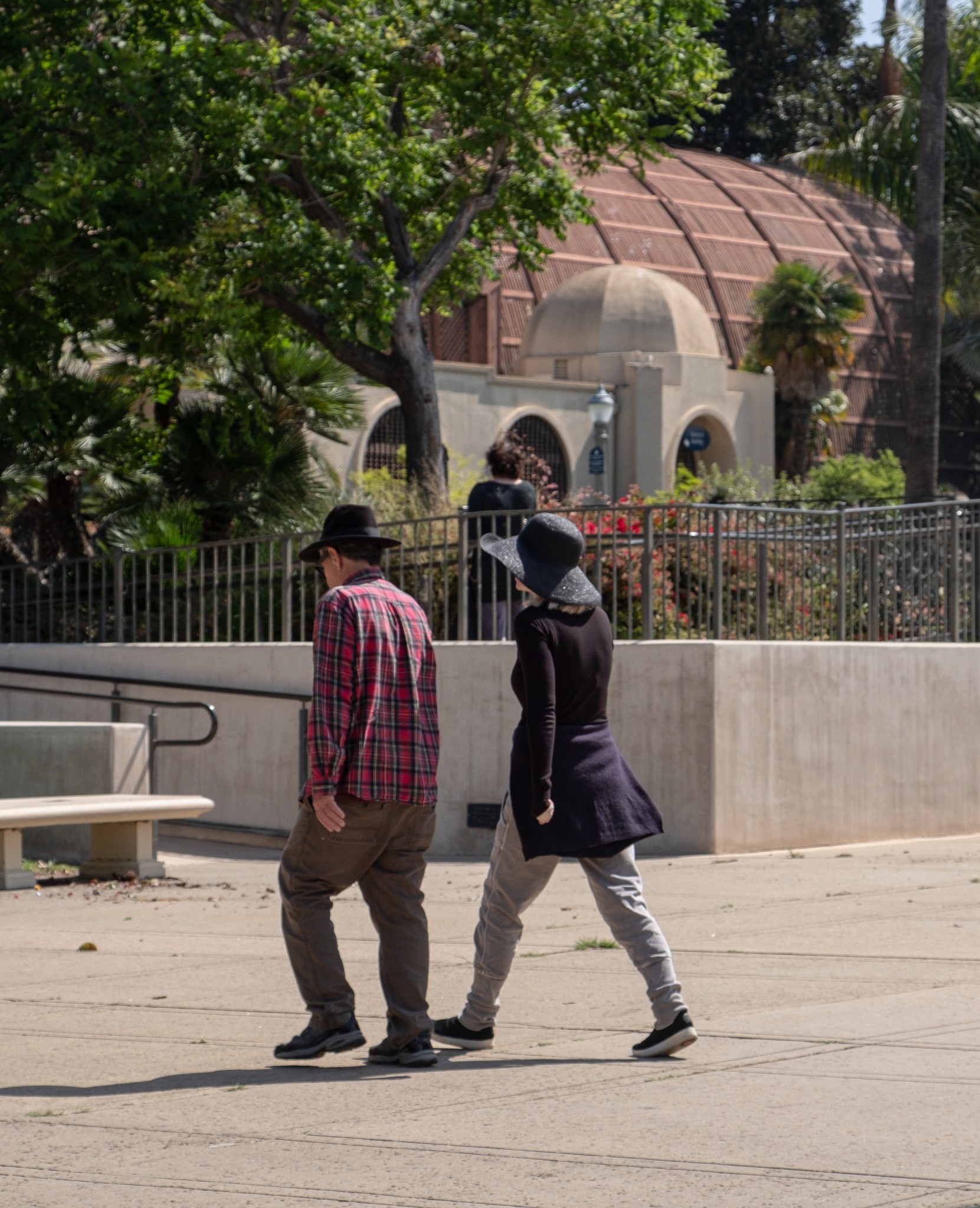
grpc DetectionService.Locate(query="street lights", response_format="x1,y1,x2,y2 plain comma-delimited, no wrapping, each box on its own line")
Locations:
585,379,618,506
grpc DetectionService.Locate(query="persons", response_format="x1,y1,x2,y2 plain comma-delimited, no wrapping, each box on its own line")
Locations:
273,505,439,1067
467,441,537,641
430,511,698,1058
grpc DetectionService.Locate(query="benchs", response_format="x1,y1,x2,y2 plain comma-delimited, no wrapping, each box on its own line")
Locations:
0,794,213,889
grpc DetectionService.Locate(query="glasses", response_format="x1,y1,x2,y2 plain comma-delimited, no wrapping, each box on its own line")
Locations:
314,549,329,576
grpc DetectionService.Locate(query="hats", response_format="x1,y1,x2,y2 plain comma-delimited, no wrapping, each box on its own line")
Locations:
479,512,602,605
297,504,401,563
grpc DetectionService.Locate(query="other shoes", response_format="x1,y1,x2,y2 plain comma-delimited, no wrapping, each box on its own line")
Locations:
631,1010,698,1058
428,1016,494,1049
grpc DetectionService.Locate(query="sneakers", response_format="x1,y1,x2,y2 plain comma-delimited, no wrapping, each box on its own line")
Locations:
273,1014,367,1061
368,1037,439,1066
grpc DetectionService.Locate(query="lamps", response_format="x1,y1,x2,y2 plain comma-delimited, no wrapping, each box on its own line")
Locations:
589,379,620,444
